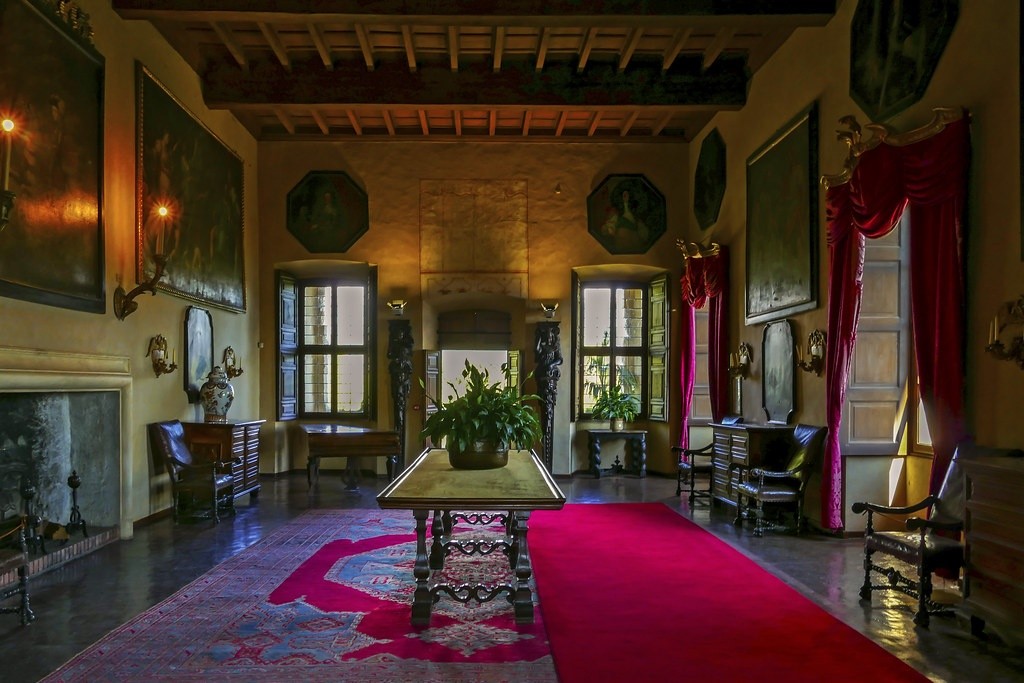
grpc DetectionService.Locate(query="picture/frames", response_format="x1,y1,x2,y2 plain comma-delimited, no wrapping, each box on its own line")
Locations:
849,0,960,123
585,171,670,256
692,126,727,232
0,0,108,316
286,168,370,255
743,98,821,325
185,307,216,402
132,61,246,314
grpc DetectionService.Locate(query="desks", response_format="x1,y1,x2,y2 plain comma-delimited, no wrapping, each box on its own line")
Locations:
377,450,566,635
296,423,401,492
588,429,648,478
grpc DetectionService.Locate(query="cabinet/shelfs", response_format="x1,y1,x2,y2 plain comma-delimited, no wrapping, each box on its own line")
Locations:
180,419,267,506
709,424,799,512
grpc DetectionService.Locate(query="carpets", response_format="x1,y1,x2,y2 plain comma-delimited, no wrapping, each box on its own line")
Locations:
38,500,934,683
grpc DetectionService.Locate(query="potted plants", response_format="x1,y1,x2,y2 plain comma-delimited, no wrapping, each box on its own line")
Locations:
406,363,556,472
592,386,637,432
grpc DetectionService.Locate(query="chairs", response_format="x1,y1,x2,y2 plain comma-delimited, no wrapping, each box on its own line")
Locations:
152,419,242,522
673,413,744,498
727,426,828,536
0,447,43,625
852,442,990,627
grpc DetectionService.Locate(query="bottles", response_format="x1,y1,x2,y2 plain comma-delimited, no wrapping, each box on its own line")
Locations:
199,366,234,422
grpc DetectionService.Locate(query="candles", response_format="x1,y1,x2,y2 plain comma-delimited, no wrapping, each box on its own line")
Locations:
795,345,802,361
3,117,15,189
730,352,738,366
988,314,998,343
155,206,167,253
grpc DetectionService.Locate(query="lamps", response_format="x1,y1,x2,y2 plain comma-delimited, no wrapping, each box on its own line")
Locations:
223,347,244,380
147,335,180,378
